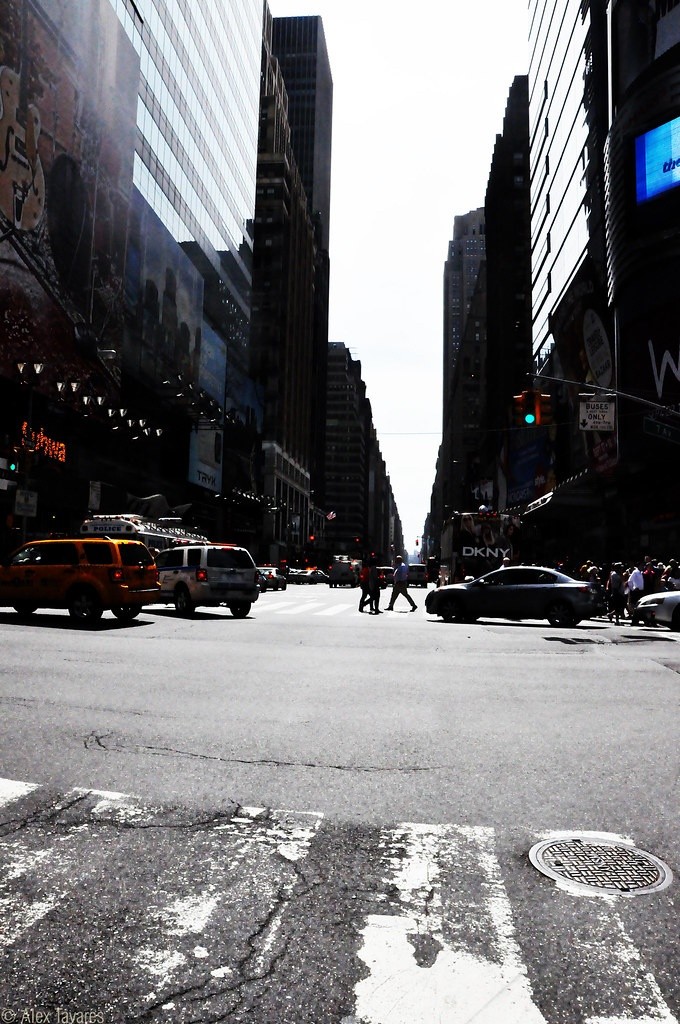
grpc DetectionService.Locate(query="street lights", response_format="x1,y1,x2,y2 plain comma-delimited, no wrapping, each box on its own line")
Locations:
70,349,116,534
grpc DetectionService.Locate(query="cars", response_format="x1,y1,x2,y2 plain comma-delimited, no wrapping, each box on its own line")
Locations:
328,560,358,588
359,567,394,589
633,590,680,633
257,567,287,592
425,566,596,627
257,570,268,593
288,569,329,584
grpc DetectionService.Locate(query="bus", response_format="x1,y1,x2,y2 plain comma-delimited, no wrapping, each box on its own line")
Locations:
439,511,522,584
80,514,211,553
425,557,440,583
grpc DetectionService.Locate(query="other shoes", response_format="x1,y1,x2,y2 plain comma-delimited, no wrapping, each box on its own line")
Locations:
410,605,417,611
360,602,383,613
615,621,624,626
384,605,393,610
607,613,612,621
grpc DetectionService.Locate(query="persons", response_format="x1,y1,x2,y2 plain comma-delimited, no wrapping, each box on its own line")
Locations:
499,557,563,583
359,558,383,615
461,515,517,547
575,555,680,628
385,556,417,612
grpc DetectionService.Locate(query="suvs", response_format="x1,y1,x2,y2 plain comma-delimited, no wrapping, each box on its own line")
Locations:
0,537,159,624
149,542,260,618
407,564,428,588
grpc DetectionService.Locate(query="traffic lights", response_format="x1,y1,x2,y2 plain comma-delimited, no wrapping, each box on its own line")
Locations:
515,391,550,429
416,539,419,546
310,536,314,544
7,456,18,474
356,538,359,542
372,551,376,560
